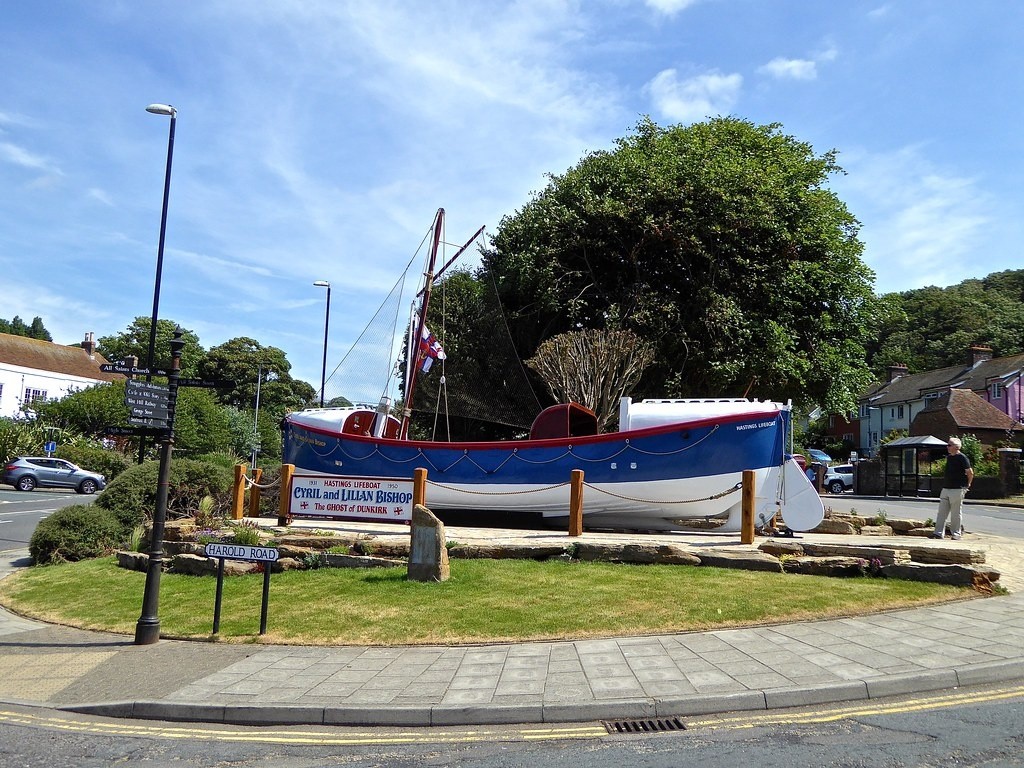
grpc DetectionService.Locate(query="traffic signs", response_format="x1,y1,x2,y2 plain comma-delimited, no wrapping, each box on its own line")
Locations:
123,378,175,430
101,363,170,377
105,426,173,436
176,376,238,389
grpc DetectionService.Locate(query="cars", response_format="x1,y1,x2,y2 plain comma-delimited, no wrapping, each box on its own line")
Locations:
2,455,107,495
805,448,832,464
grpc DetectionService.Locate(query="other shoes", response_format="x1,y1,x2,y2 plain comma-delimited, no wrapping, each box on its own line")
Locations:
951,535,959,540
928,533,943,539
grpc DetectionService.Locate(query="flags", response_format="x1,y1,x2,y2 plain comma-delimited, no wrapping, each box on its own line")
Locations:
412,312,447,373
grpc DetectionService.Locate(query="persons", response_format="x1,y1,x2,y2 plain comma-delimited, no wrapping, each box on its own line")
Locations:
805,465,817,489
928,438,973,540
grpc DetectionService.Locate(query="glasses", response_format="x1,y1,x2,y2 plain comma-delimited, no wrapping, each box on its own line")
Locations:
948,444,951,447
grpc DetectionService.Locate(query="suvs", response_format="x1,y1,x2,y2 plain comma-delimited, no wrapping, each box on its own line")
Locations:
822,464,854,494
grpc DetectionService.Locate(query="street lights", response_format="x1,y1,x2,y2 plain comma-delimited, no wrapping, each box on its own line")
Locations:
313,279,331,408
137,103,179,464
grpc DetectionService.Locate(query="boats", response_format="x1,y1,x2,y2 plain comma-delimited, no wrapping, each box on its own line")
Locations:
271,208,824,532
